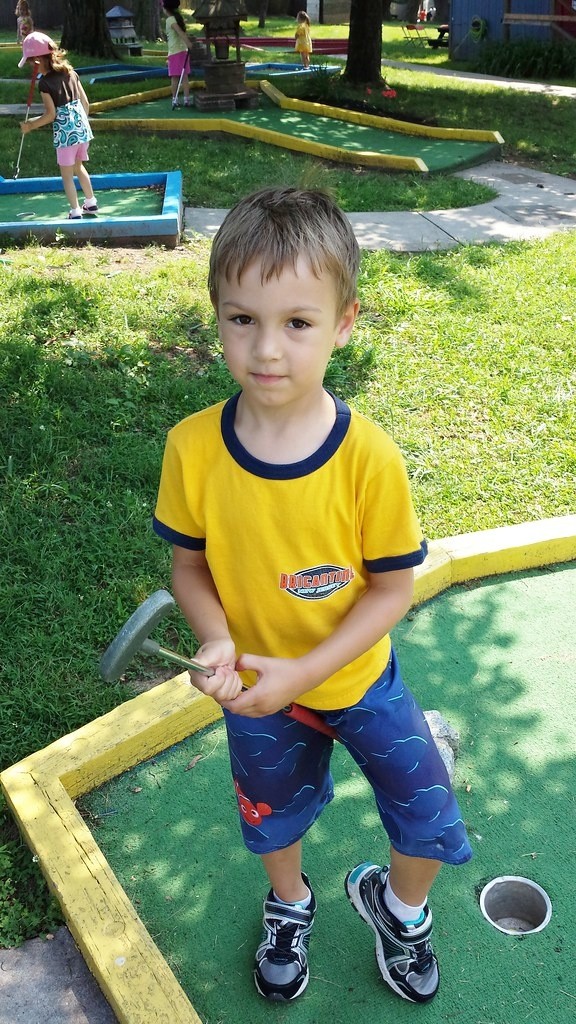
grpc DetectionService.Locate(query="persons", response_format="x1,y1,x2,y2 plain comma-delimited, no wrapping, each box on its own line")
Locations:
15,0,34,46
294,11,312,70
147,189,473,1003
18,31,99,220
160,0,193,108
420,5,437,21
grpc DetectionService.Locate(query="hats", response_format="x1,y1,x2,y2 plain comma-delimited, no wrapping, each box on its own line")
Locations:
18,32,55,67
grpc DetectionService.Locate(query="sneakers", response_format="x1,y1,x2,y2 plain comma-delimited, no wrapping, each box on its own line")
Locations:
253,871,317,1002
343,860,440,1004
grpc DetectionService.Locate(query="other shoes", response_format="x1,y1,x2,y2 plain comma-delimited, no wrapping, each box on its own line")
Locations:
82,204,98,214
172,100,180,106
69,213,81,219
184,101,190,106
